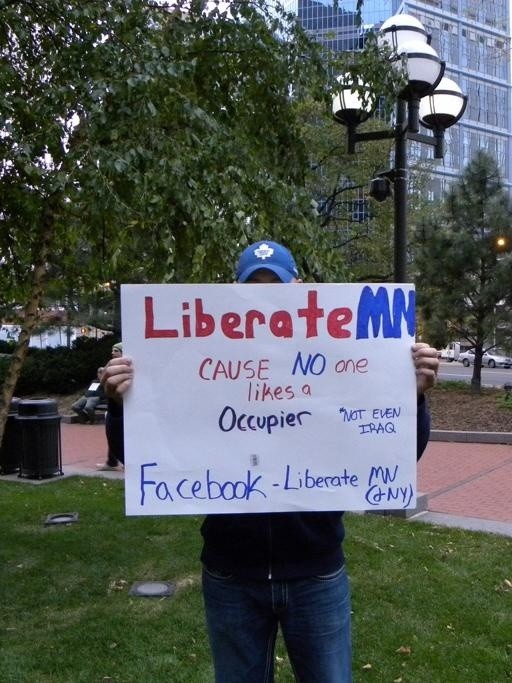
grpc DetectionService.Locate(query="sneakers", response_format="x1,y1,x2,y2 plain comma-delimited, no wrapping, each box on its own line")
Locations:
95,463,122,471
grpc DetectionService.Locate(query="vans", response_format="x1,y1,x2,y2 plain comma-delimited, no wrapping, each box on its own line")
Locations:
440,342,460,362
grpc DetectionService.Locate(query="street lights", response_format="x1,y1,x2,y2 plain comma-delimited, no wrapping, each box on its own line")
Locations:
330,12,470,284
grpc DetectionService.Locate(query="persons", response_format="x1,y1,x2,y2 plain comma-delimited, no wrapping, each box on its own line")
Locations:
102,240,439,682
96,343,124,471
70,378,102,425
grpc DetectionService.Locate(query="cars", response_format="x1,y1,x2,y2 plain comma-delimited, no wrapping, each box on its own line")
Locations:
458,347,512,370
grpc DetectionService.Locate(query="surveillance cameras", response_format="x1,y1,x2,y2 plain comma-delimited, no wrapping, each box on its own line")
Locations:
369,177,392,202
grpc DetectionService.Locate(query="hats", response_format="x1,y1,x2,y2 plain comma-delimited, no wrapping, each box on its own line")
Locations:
235,240,296,282
112,340,122,353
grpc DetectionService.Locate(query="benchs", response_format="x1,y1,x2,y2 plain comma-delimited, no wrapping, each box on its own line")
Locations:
82,396,109,423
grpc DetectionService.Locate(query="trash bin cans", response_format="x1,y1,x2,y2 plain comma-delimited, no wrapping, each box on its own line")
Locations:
1,395,63,480
